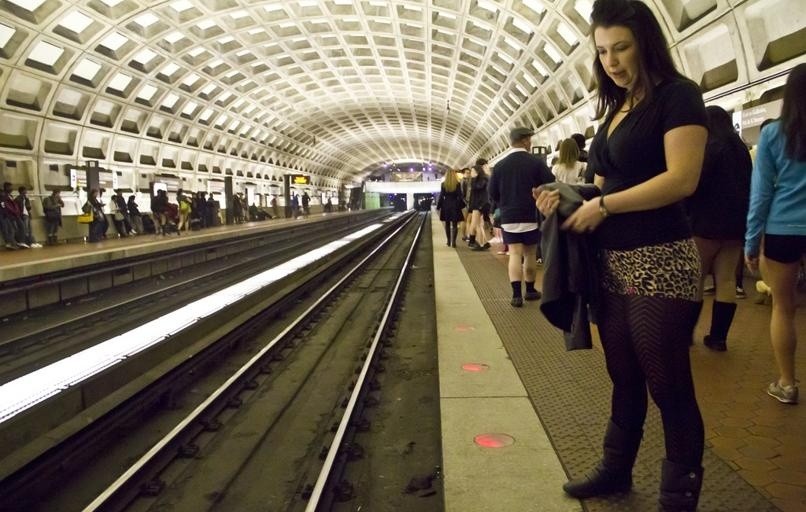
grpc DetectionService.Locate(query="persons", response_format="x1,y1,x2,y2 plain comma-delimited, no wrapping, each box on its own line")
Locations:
537,132,589,265
437,159,509,255
0,183,360,252
703,247,747,299
488,125,557,308
689,106,753,350
744,62,804,404
533,1,711,511
748,119,773,307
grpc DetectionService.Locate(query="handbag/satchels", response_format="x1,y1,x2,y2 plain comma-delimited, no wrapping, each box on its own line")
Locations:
179,200,192,215
456,191,467,211
114,209,125,222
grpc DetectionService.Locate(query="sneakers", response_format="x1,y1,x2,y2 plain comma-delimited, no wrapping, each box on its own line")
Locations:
524,288,543,301
766,377,802,407
735,286,748,300
112,228,138,239
462,234,510,257
3,234,61,252
702,283,718,297
509,296,525,308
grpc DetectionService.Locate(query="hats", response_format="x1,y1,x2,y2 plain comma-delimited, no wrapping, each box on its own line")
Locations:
508,125,537,144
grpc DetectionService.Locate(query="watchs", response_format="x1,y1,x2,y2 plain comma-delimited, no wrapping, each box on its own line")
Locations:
599,193,612,219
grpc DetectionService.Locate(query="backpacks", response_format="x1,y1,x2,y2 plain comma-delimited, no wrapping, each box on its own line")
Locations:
81,198,91,213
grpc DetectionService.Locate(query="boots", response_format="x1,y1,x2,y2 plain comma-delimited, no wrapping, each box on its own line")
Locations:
444,226,452,247
689,300,707,347
703,297,739,353
561,416,645,501
451,227,459,249
